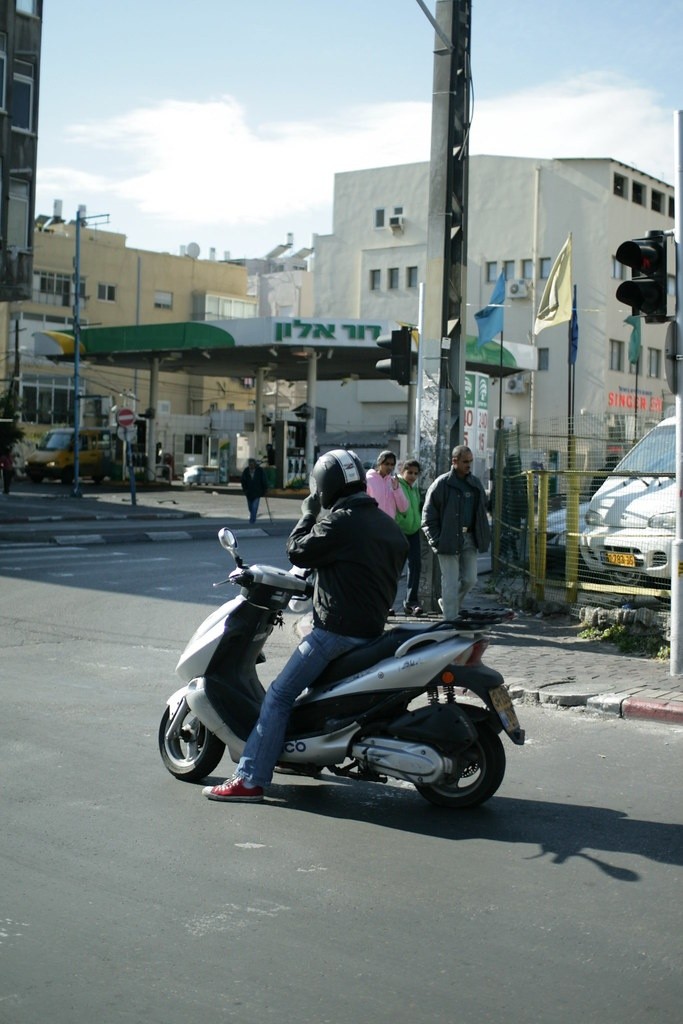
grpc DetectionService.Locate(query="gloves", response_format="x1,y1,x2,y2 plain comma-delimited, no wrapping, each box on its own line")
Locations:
300,493,322,516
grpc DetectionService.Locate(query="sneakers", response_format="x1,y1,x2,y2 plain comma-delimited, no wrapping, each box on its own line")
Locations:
202,773,264,803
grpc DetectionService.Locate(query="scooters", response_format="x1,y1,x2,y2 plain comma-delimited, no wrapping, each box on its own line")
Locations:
159,527,526,814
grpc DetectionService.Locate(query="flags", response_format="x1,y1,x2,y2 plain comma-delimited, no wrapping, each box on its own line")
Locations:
623,315,641,365
568,285,579,365
474,271,506,353
533,235,574,337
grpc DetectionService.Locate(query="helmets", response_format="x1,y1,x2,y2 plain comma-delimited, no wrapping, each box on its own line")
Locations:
311,449,367,508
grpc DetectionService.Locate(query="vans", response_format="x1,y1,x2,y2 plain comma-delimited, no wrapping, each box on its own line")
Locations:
579,411,674,581
25,427,111,485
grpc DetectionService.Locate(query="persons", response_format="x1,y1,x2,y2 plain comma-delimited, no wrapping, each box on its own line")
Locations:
240,458,269,524
366,445,624,620
201,449,409,803
0,446,13,496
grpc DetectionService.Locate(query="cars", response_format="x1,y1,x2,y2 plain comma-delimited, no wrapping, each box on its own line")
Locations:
531,499,592,576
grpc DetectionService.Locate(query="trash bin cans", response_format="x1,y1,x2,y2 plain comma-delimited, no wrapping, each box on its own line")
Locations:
265,467,277,488
111,464,122,481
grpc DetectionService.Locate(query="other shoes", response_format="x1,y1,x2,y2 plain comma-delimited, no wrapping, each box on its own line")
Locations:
388,608,395,616
438,598,444,612
403,604,425,615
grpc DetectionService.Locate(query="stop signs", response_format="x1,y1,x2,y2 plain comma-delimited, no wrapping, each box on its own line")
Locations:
117,408,135,428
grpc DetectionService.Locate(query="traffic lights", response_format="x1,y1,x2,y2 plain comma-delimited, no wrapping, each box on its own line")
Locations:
615,231,668,327
376,328,412,386
